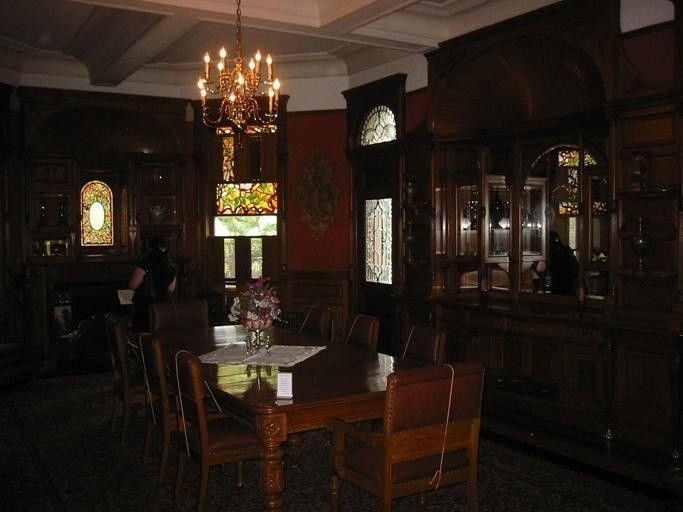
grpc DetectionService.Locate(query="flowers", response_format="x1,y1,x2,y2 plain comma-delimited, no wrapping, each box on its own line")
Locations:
228,275,288,331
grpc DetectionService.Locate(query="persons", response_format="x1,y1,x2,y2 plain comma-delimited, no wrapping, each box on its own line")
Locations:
534,231,577,294
127,234,179,335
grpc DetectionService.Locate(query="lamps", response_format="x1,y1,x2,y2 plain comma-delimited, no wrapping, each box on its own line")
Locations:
196,0,281,151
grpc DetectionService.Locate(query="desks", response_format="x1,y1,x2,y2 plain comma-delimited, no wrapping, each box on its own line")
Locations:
138,323,436,509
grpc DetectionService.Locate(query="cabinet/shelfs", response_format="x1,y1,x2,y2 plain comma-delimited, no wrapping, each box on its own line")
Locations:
342,2,682,488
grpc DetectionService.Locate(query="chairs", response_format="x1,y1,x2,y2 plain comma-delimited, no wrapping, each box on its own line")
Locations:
169,346,264,510
297,309,331,337
151,294,208,334
108,320,152,442
325,359,484,510
398,321,450,363
319,311,381,448
132,331,189,482
